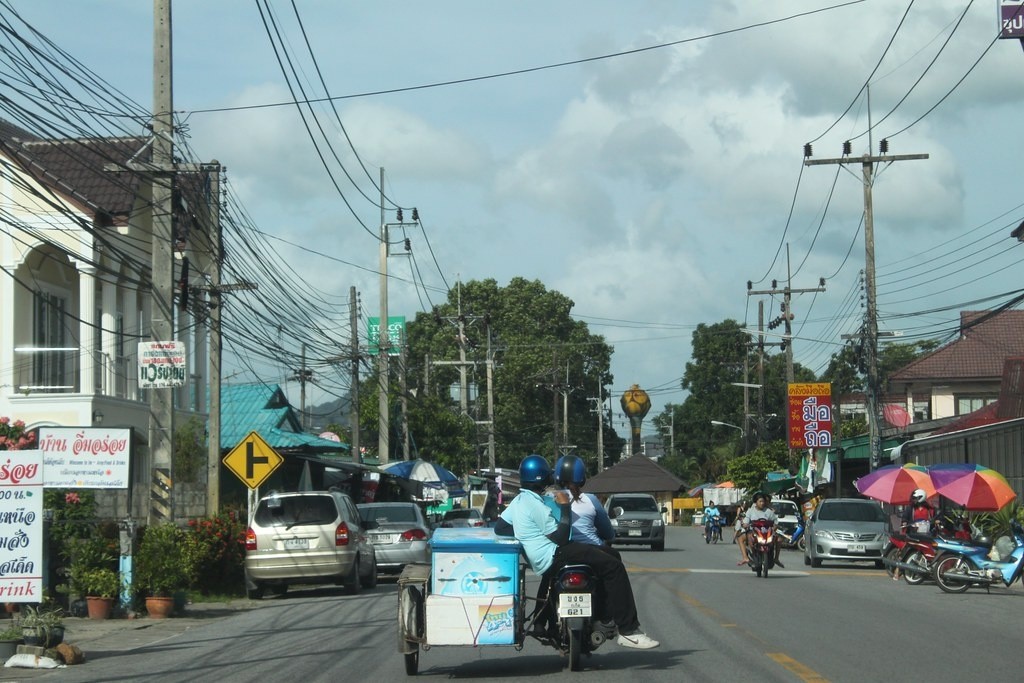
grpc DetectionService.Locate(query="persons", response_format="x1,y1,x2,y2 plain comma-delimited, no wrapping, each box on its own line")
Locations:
532,455,623,632
495,455,659,648
732,492,785,569
892,489,936,580
702,501,724,541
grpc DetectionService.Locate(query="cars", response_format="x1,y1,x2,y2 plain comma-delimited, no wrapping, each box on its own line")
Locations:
805,498,892,569
602,493,667,551
355,501,441,575
771,499,800,535
442,508,489,528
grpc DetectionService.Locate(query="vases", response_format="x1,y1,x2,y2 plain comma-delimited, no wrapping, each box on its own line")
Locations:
197,568,222,596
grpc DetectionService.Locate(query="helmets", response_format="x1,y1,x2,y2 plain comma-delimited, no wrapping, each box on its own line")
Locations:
556,455,587,484
973,532,993,547
518,454,555,482
909,488,927,504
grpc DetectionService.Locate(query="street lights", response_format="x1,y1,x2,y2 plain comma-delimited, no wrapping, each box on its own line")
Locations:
710,421,749,455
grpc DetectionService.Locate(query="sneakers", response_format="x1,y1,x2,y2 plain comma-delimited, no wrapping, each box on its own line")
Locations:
617,629,660,649
592,618,616,632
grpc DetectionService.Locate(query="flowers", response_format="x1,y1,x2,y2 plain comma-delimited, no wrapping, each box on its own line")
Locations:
0,416,249,614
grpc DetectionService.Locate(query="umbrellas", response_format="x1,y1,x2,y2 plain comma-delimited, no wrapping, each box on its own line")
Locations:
852,462,1016,513
378,458,460,500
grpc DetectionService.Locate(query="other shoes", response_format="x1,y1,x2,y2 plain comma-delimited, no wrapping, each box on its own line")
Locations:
533,624,547,633
736,559,752,566
774,559,785,568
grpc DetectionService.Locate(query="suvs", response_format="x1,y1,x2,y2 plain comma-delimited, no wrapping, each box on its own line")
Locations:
244,490,377,599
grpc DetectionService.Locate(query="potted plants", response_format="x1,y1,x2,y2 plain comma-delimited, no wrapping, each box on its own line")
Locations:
132,522,208,619
19,602,66,647
0,626,24,657
81,567,123,621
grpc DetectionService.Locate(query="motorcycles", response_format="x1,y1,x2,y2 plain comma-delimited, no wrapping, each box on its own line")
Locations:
927,505,1023,593
738,512,785,577
702,509,724,545
881,504,971,586
773,511,805,552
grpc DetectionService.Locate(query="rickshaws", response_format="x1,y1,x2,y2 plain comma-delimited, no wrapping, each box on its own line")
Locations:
398,507,625,676
